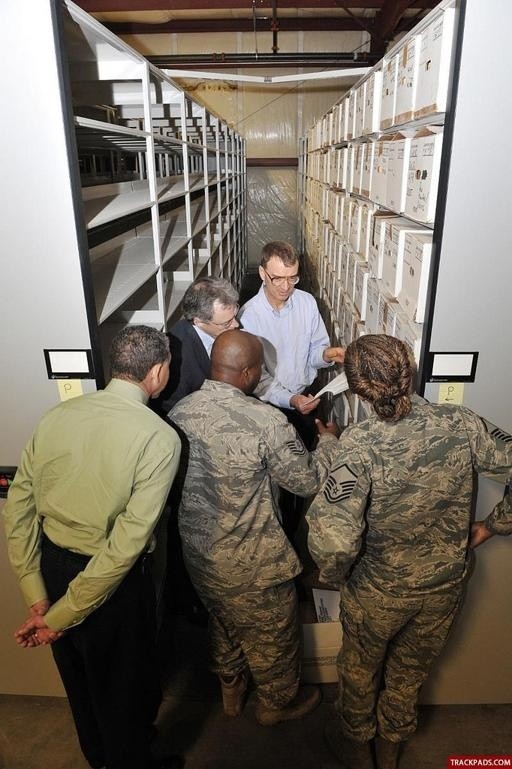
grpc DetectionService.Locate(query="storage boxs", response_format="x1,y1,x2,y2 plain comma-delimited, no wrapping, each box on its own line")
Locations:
290,0,457,434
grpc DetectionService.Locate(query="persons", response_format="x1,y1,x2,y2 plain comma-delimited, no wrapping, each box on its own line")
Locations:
151,275,239,424
304,334,512,769
2,325,182,769
166,329,340,725
236,240,346,535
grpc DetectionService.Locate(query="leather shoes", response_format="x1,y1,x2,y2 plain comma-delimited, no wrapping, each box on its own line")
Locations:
89,724,156,767
158,755,183,768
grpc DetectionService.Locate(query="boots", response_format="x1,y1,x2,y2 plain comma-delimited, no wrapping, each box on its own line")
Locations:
254,683,321,726
220,674,250,718
324,720,376,769
373,733,401,768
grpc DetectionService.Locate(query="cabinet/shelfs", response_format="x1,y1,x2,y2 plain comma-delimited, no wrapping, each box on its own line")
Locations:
51,0,249,385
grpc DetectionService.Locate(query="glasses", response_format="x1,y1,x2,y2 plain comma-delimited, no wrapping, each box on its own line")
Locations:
265,270,300,287
210,302,240,328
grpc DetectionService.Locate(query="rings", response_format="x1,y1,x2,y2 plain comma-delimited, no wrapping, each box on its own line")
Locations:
34,633,37,638
